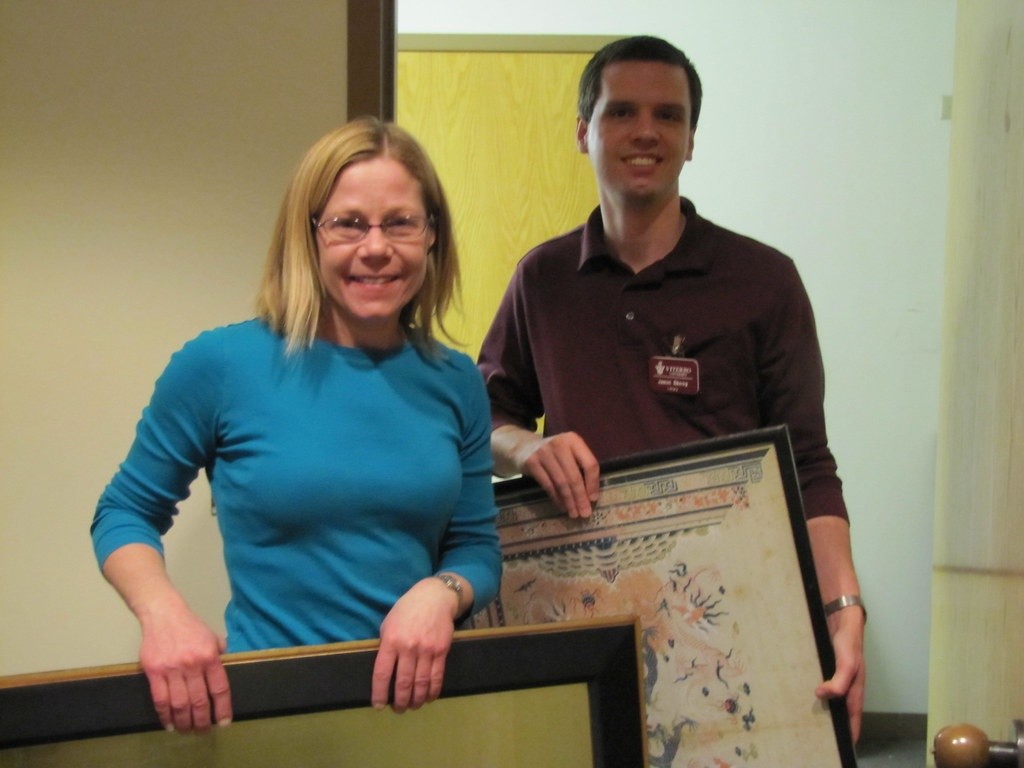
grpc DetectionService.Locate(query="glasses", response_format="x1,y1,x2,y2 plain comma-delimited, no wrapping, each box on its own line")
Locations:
311,212,434,240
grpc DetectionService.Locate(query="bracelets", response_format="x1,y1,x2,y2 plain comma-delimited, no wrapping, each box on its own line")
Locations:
819,588,868,630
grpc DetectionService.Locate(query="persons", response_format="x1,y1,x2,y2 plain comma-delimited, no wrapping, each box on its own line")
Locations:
473,34,869,750
89,114,503,739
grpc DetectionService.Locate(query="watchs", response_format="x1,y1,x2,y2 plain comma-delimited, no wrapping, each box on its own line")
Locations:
433,571,467,615
